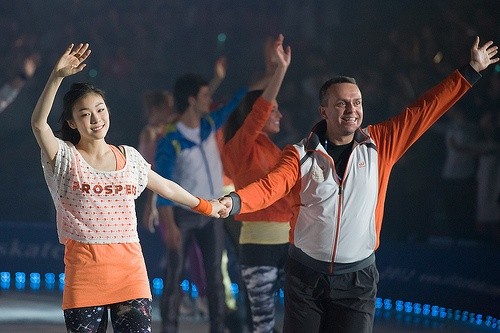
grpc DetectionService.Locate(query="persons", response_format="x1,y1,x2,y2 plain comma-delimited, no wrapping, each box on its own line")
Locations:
0,54,38,115
217,37,500,333
138,35,292,333
32,43,226,333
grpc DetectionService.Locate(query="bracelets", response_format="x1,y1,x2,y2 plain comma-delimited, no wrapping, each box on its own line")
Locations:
192,197,212,218
